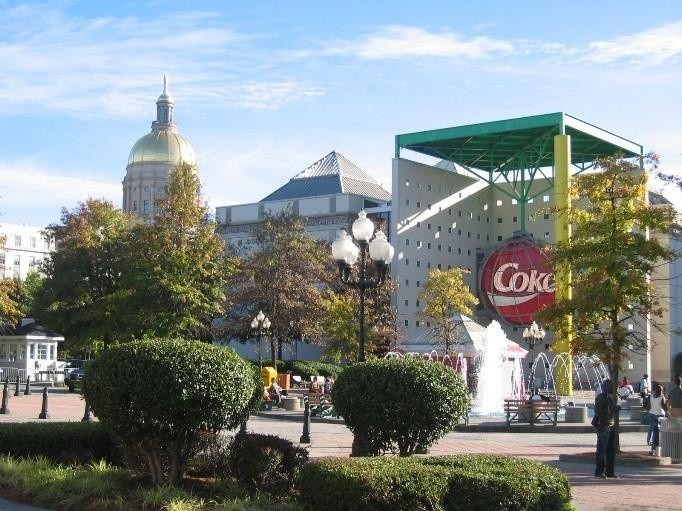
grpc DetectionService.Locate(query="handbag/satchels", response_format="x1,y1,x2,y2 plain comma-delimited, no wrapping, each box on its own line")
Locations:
665,410,671,417
591,414,608,430
644,397,650,409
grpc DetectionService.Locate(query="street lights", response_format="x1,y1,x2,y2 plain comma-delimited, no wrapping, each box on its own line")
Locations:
523,320,545,400
251,310,272,373
332,208,395,362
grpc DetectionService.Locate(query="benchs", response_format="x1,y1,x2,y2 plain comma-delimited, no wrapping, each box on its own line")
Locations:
304,393,339,419
503,399,564,427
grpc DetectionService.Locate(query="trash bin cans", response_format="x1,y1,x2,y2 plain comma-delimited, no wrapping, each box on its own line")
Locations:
279,373,291,389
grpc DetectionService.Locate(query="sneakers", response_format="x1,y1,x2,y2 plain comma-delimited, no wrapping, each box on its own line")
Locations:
606,474,618,478
595,472,607,478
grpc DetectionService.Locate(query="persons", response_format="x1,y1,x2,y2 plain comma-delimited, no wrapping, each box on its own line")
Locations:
591,380,621,479
617,374,682,446
262,376,332,406
